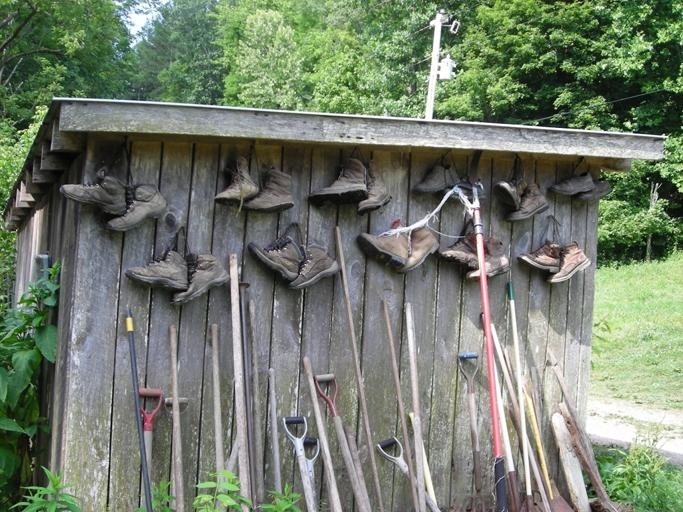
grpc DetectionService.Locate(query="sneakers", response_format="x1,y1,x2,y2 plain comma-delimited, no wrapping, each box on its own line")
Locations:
445,177,486,200
413,163,460,194
549,173,594,196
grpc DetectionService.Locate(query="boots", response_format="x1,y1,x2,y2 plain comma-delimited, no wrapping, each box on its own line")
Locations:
247,223,304,282
170,249,229,306
357,160,393,216
356,226,410,270
494,156,527,212
59,175,130,214
466,238,511,280
214,155,259,219
547,241,591,282
506,183,550,222
396,225,440,273
242,169,294,213
517,238,560,273
125,227,188,291
105,186,169,233
286,242,340,290
309,156,366,207
434,232,489,270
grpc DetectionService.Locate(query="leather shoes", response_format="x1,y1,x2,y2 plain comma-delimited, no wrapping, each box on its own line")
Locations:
571,179,612,202
